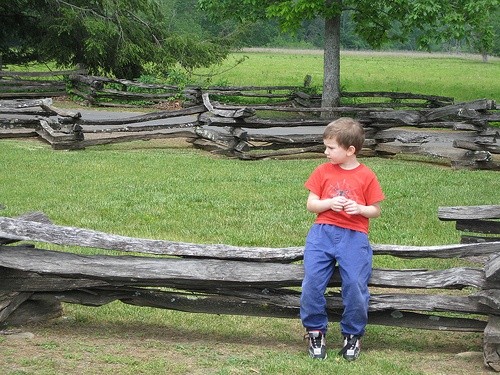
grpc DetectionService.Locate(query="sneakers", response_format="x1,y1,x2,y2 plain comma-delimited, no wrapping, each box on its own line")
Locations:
341,332,361,361
306,330,327,361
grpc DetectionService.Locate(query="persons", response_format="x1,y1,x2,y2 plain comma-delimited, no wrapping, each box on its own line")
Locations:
299,117,385,362
